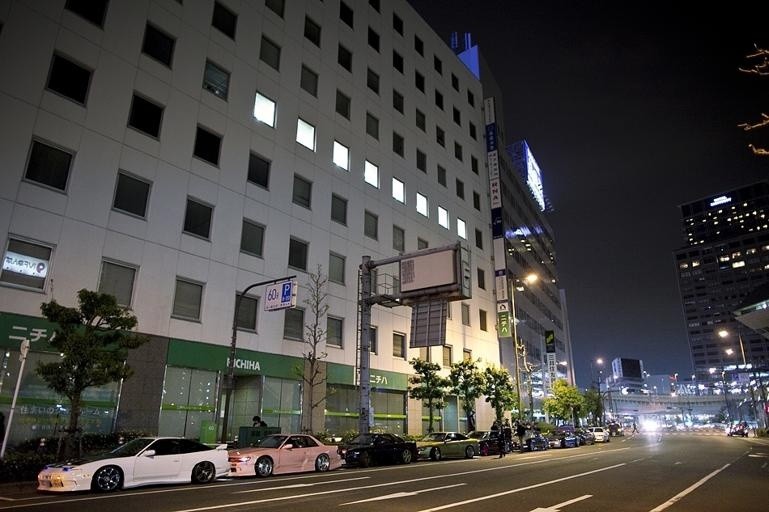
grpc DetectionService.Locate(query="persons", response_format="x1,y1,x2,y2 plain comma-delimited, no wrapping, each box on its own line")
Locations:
632,421,640,435
253,415,267,435
490,418,524,445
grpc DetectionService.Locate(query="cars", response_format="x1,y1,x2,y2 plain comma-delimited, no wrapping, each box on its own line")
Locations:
467,429,513,455
510,431,550,451
605,424,624,435
548,425,611,448
340,431,419,469
725,424,749,436
416,431,481,460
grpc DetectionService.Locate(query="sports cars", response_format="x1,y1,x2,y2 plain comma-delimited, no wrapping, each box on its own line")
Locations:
227,432,344,479
36,437,230,494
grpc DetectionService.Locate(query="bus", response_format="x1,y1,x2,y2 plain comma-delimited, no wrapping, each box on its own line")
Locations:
622,416,634,429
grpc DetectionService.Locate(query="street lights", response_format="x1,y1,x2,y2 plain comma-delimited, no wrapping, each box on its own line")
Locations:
723,346,740,383
507,272,539,419
710,367,734,421
587,355,604,387
718,326,758,421
